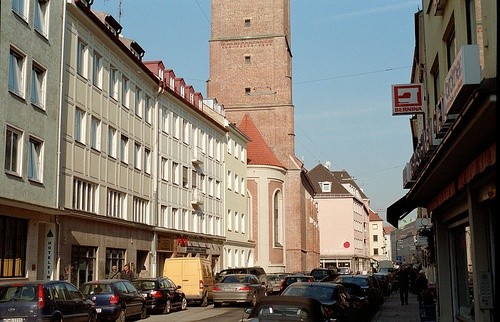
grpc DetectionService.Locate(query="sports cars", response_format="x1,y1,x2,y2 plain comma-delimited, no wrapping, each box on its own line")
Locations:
239,295,329,322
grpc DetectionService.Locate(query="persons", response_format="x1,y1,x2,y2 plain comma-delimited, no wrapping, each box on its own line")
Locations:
110,265,151,279
397,265,426,305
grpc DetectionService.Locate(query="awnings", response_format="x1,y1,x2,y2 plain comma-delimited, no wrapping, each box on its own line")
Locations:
387,196,416,229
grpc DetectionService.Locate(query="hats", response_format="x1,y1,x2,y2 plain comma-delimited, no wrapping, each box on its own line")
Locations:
112,266,117,270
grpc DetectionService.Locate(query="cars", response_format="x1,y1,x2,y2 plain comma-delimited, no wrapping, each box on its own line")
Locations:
133,276,187,314
279,276,316,295
0,280,97,322
212,274,267,306
280,282,358,321
80,279,147,322
334,274,381,310
267,260,399,302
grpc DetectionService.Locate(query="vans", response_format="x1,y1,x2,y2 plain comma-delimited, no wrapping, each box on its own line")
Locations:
215,267,267,285
163,257,215,306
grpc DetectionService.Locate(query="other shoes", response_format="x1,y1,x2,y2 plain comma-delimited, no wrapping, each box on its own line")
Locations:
400,303,404,305
406,302,409,306
